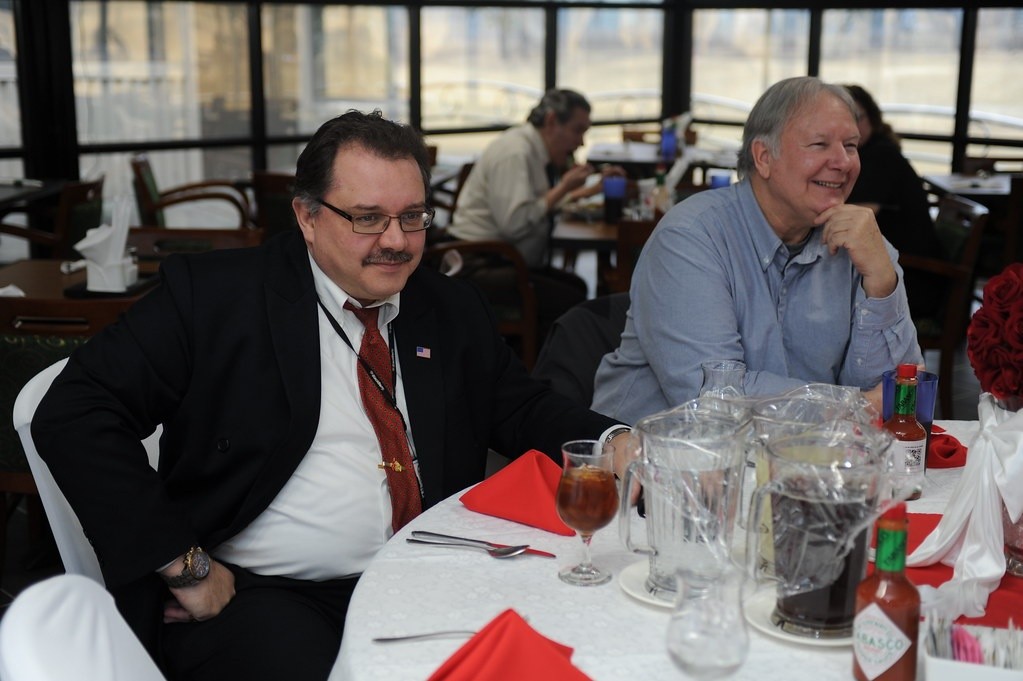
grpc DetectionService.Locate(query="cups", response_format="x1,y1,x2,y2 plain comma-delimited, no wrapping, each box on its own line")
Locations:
604,177,625,222
713,175,729,189
622,410,753,601
752,397,862,583
767,430,882,640
882,372,938,475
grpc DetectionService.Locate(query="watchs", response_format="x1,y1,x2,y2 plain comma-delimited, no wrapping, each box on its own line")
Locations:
168,546,212,588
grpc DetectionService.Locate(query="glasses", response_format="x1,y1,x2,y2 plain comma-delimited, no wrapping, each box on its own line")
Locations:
857,112,867,123
309,196,435,234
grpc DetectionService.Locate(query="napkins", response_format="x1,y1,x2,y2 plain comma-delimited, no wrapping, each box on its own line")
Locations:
868,412,970,470
459,451,581,537
428,609,590,681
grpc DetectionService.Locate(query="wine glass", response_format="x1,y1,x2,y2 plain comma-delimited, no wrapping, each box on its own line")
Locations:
556,440,620,585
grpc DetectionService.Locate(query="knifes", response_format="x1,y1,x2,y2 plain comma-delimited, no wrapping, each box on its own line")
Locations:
411,531,556,557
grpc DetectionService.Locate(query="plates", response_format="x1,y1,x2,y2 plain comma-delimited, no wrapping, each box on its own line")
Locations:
743,586,852,646
562,209,605,218
619,560,675,608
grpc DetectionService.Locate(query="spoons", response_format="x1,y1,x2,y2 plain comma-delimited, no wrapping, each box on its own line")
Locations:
406,539,529,559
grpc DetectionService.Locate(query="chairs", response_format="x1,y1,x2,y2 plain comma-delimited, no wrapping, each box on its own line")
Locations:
885,193,990,414
0,359,180,681
126,147,257,259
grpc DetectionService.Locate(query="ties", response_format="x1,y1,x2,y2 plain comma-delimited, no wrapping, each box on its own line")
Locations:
547,163,557,250
344,301,422,534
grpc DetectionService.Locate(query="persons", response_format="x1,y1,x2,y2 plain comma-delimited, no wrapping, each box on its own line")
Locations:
28,108,737,680
588,76,944,425
446,89,625,324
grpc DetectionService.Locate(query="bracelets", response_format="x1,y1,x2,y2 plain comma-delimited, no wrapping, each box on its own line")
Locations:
602,427,634,446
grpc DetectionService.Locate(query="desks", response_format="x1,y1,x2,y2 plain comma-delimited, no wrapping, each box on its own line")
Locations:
547,212,634,303
2,260,163,342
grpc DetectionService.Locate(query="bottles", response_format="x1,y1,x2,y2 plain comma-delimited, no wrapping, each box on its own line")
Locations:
853,528,921,680
697,359,747,412
655,163,665,221
662,128,675,158
881,363,927,501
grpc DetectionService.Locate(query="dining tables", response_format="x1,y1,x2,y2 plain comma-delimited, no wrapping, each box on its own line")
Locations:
326,419,1022,681
